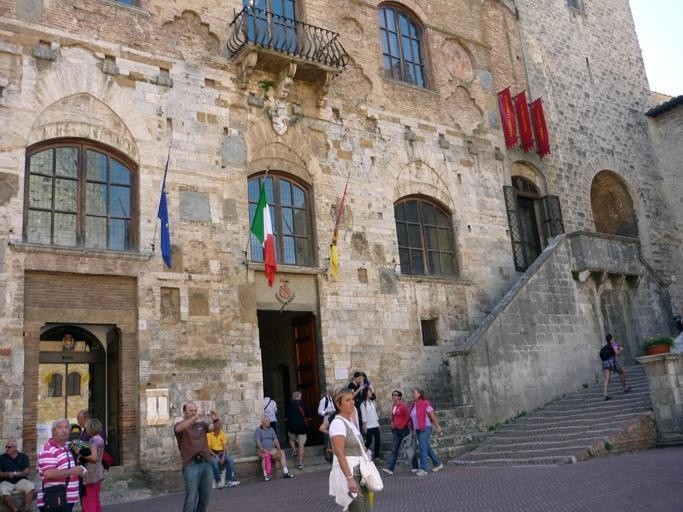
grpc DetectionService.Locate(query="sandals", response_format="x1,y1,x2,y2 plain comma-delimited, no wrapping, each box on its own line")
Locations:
297,463,304,469
283,472,293,478
264,475,269,482
293,450,297,456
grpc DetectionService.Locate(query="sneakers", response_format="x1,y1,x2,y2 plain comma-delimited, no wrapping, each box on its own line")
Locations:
410,468,419,473
416,469,428,476
382,468,393,476
216,481,224,488
432,462,443,472
226,481,240,487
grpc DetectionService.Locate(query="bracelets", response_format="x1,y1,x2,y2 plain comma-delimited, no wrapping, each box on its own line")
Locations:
212,418,219,422
346,474,354,480
191,415,199,426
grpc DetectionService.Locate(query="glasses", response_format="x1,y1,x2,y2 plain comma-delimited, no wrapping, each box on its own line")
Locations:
5,446,16,449
391,394,399,397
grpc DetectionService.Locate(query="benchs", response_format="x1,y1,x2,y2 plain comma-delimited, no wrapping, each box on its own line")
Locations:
8,489,27,496
233,456,262,464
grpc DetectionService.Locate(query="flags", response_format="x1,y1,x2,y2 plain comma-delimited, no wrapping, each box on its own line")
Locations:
250,180,277,288
329,187,348,283
157,183,172,270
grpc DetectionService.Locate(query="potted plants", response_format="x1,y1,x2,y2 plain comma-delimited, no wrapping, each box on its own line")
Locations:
643,336,675,355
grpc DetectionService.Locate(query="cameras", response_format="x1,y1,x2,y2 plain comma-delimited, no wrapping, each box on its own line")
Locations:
205,410,212,416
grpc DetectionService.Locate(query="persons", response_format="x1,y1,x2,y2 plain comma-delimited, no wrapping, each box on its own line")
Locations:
285,390,308,470
0,439,35,512
173,402,224,512
317,372,384,462
263,390,279,435
381,389,420,475
600,334,632,399
206,422,241,490
253,415,295,481
327,384,384,512
409,386,444,477
35,408,105,511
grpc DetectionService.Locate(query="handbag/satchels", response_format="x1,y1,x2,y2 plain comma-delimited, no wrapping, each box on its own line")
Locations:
102,452,113,471
360,461,384,492
44,484,67,512
401,434,418,464
